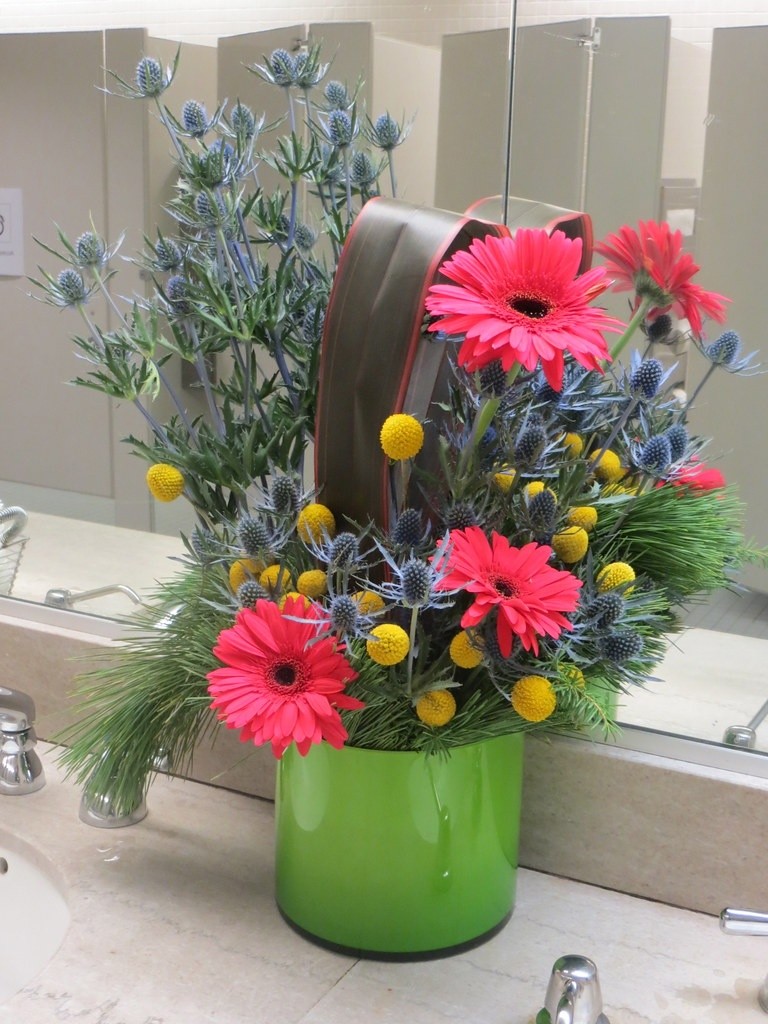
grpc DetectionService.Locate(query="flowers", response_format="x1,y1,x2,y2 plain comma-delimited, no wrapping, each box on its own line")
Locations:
22,33,768,818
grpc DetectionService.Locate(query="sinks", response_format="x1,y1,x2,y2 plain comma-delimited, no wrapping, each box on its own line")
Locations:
0,824,76,1012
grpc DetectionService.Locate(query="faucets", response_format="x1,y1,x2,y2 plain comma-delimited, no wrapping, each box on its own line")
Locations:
44,582,142,612
0,684,47,798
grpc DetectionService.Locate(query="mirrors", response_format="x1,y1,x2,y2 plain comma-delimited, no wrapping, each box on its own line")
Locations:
0,0,768,758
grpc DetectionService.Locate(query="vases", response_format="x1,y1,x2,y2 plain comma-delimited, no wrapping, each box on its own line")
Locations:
271,732,526,962
